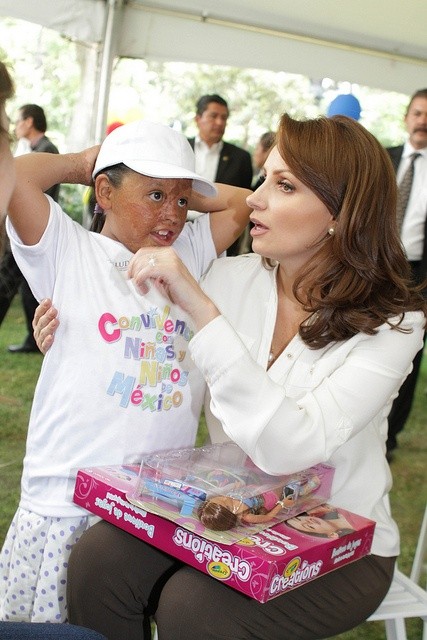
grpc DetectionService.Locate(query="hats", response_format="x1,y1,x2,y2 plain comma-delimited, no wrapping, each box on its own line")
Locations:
90,121,217,199
327,93,361,121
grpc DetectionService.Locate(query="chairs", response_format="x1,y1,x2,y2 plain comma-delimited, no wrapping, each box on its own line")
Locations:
367,506,427,640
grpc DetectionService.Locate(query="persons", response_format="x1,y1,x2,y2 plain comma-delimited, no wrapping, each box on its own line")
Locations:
32,115,425,640
197,471,322,532
0,120,255,640
0,51,279,354
373,88,426,464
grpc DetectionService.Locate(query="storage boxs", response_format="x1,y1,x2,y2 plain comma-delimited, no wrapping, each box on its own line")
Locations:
72,457,376,604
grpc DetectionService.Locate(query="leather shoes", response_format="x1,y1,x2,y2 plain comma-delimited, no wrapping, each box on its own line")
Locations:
8,342,38,353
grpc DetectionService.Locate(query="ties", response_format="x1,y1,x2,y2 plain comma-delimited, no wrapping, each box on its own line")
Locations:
396,152,420,236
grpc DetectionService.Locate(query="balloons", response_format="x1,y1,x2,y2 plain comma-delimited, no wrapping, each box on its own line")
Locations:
327,93,362,123
